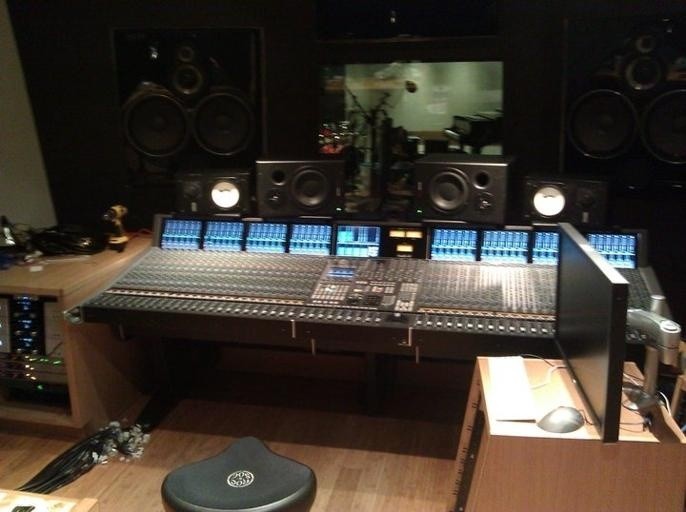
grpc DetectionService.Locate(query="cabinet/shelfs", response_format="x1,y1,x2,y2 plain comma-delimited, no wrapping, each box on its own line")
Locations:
0,230,156,441
446,356,686,512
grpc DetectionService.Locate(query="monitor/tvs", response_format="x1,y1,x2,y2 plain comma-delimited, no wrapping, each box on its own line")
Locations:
552,220,682,443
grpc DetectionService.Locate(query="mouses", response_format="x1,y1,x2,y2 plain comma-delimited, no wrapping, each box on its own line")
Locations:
538,405,585,435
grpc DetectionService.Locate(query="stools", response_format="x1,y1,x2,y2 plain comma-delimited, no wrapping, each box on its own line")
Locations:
162,434,315,511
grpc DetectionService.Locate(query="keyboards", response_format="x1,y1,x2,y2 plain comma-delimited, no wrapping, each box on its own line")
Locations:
482,353,538,423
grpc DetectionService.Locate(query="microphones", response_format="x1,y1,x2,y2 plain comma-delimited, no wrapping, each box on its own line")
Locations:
391,79,418,108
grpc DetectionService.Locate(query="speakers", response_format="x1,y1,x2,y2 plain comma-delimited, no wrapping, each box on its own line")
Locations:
411,156,510,233
563,1,686,224
107,27,267,221
254,158,349,218
